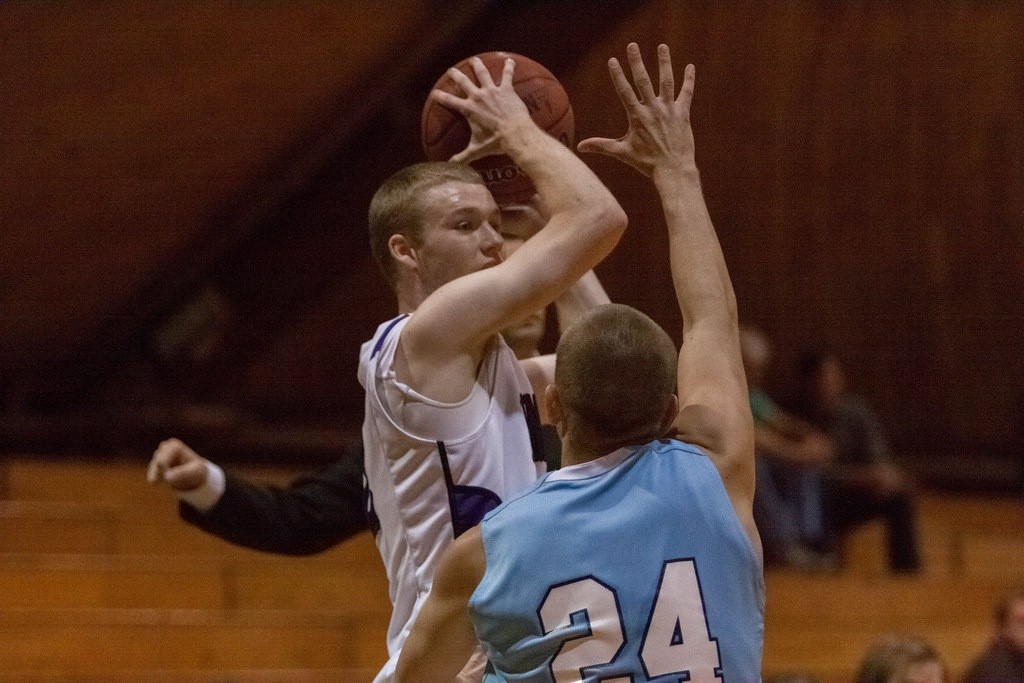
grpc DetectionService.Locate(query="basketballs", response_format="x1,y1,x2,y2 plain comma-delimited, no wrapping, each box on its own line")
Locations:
417,51,579,204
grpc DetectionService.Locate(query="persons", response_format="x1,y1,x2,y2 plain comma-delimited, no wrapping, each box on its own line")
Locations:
148,42,764,683
856,596,1024,683
734,324,920,574
